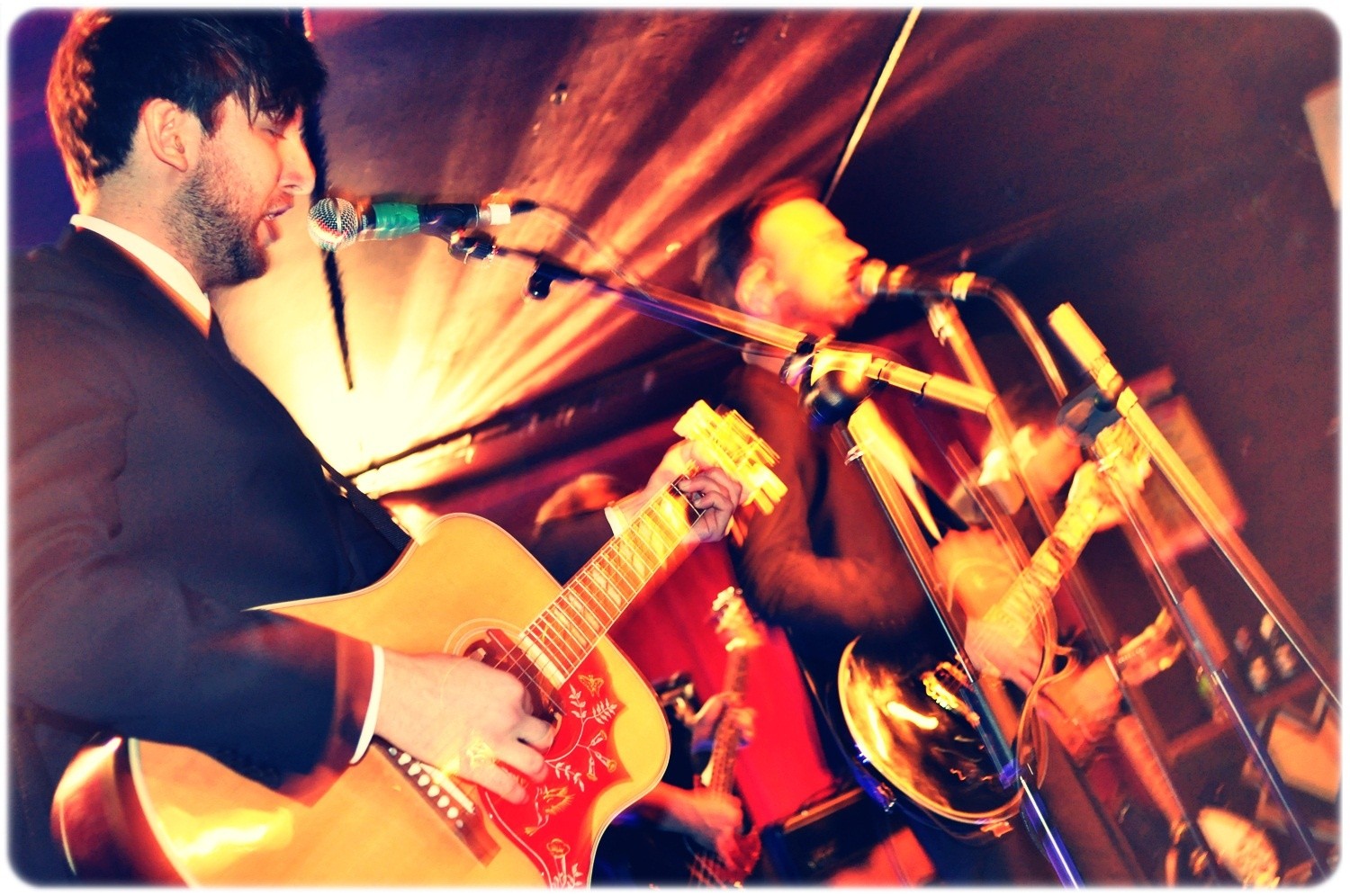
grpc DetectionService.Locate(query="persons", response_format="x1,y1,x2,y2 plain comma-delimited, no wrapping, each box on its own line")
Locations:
534,475,755,890
11,7,740,890
715,178,1145,890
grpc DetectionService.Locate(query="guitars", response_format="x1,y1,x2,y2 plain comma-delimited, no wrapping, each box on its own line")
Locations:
599,586,769,887
828,418,1154,845
45,399,791,886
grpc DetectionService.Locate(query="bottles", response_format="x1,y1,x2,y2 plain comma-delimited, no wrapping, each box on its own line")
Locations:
1234,624,1277,695
1258,612,1303,680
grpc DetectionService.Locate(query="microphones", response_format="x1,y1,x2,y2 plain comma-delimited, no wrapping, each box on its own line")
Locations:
854,258,995,301
303,197,513,250
